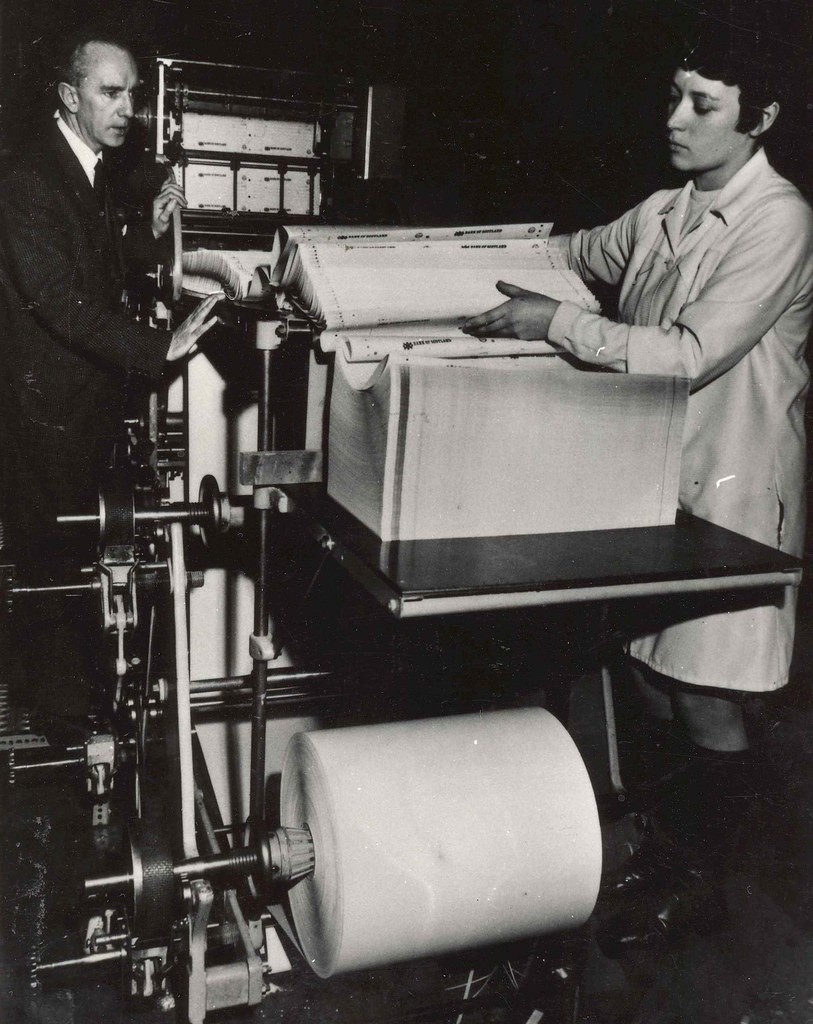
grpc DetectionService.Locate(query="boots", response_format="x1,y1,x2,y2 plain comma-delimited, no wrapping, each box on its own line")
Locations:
596,737,751,959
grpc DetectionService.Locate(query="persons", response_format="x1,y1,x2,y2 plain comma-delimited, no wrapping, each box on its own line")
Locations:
462,12,813,957
1,23,228,718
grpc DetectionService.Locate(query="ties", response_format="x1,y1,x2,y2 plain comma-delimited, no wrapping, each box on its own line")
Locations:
93,158,104,231
